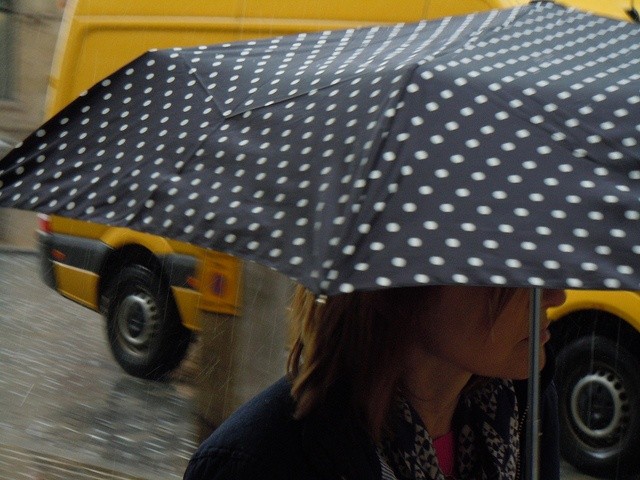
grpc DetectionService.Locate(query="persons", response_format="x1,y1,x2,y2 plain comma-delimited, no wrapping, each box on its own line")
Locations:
183,282,566,480
